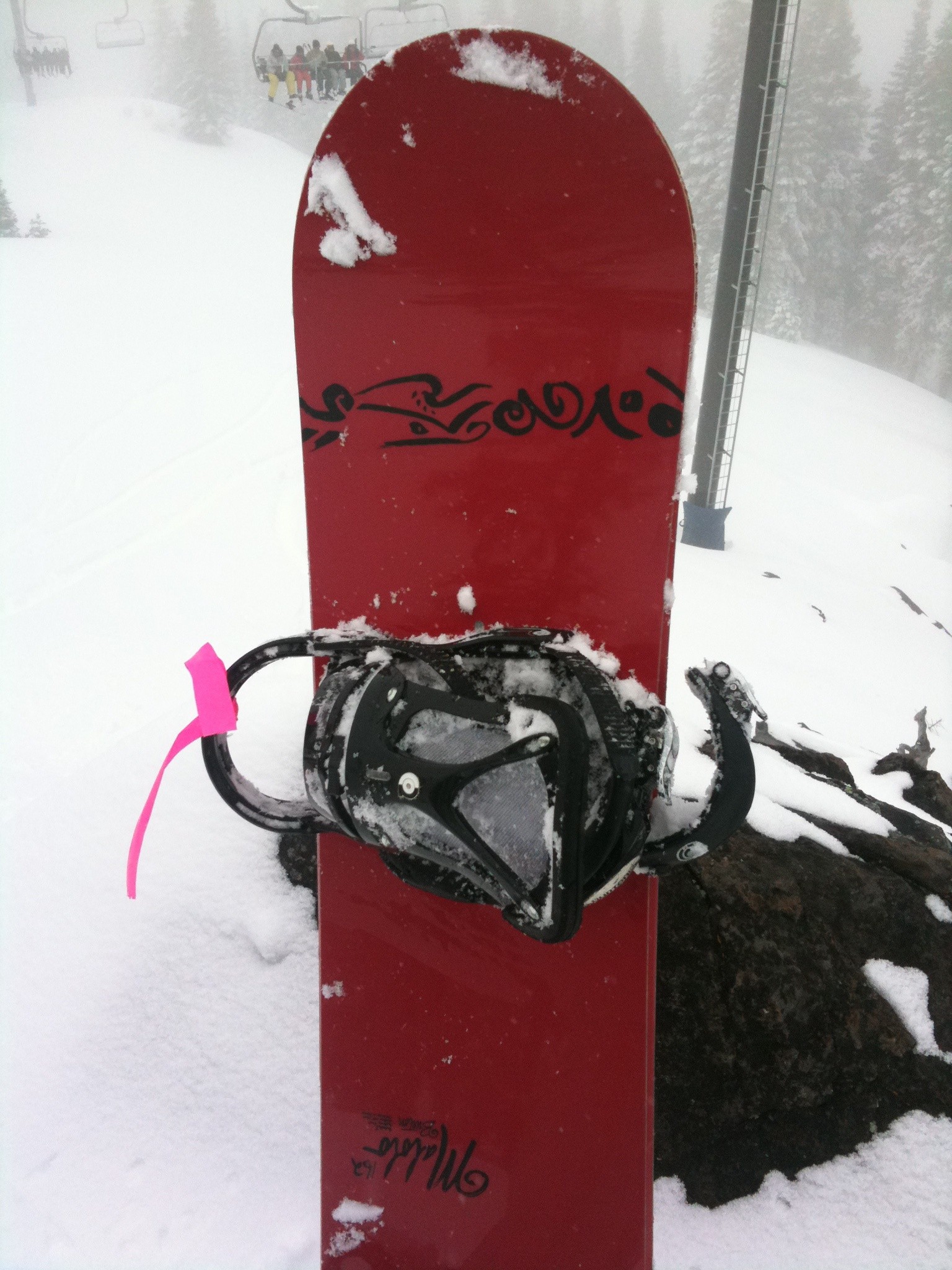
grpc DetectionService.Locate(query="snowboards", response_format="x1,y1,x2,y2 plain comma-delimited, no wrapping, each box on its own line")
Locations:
293,27,695,1270
